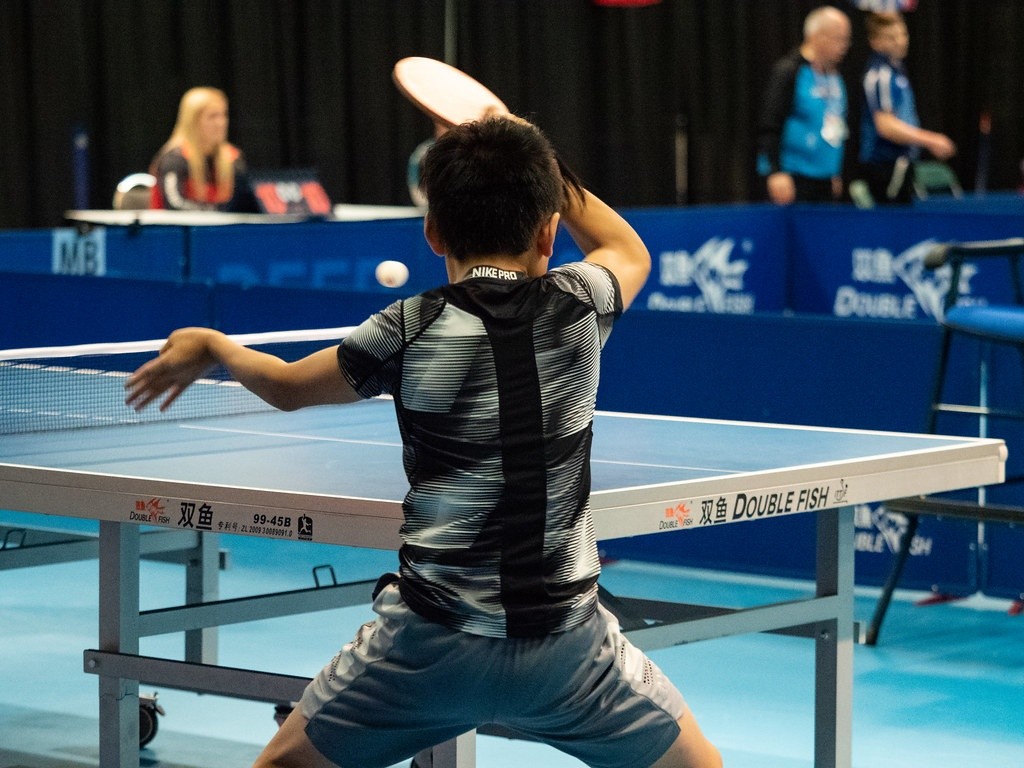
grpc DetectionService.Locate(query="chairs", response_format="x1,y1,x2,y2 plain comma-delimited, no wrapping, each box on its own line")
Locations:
112,173,162,213
860,236,1024,648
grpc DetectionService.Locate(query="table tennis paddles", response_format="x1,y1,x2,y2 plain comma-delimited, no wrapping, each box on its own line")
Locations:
395,57,512,130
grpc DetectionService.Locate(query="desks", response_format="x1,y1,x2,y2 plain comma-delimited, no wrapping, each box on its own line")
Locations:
0,360,1010,768
65,202,430,230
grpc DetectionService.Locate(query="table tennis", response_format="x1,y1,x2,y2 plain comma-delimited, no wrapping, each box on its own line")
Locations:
375,261,408,288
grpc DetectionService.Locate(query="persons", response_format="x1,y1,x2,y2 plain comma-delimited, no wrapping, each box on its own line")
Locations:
147,87,267,213
746,5,955,205
123,104,725,768
